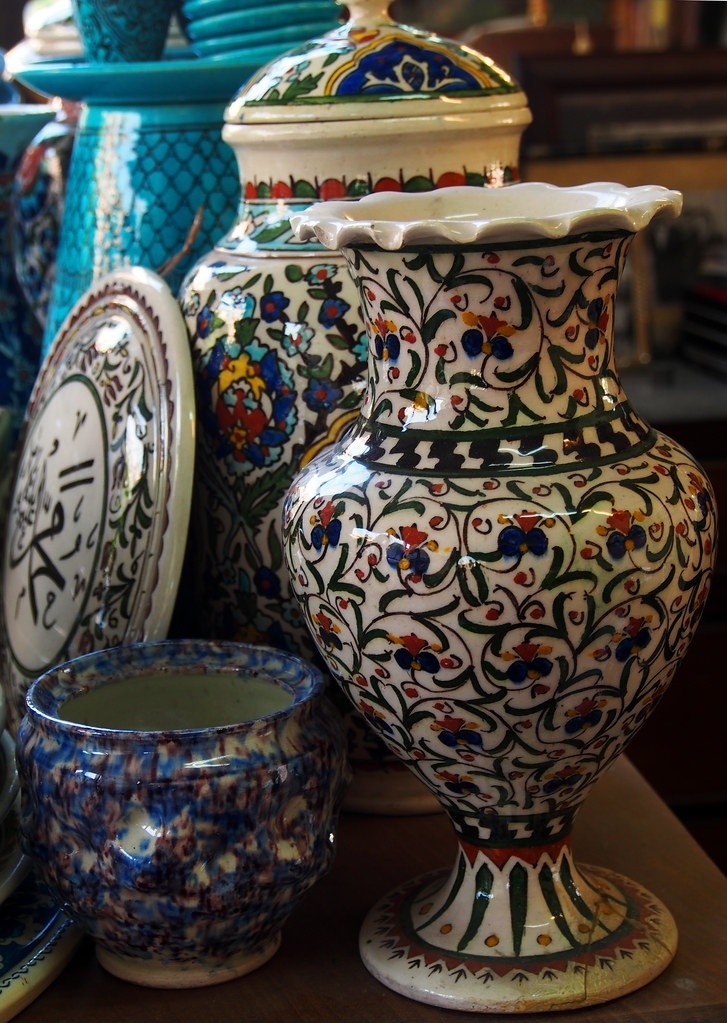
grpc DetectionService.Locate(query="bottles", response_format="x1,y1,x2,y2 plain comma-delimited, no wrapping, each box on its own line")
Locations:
286,181,718,1014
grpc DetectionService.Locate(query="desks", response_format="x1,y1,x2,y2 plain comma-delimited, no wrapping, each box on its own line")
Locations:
0,738,727,1022
